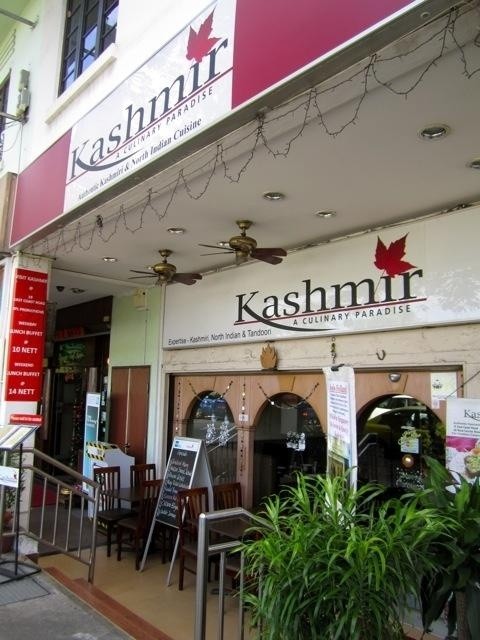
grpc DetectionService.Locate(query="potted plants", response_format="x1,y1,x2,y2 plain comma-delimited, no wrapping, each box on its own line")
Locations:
0,451,27,554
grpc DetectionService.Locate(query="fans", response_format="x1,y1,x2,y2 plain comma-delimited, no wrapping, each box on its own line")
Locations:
128,250,202,286
198,220,287,265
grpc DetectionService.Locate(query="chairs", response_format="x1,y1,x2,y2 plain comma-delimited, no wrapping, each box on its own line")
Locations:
93,463,293,614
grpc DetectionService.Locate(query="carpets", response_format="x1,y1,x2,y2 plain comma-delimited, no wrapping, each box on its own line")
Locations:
0,552,136,640
31,479,60,507
0,557,51,606
